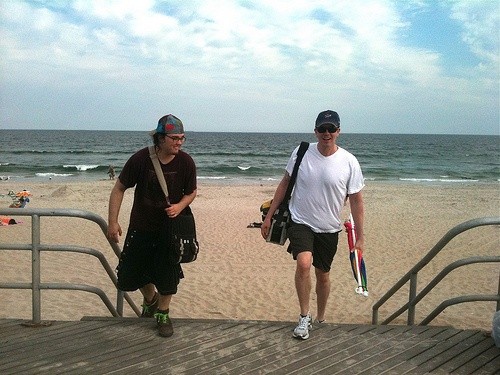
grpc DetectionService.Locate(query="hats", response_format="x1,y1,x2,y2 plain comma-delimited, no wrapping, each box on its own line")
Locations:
316,110,340,128
156,114,184,134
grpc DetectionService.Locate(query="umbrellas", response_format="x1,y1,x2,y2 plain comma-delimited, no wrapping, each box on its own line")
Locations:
344,212,369,297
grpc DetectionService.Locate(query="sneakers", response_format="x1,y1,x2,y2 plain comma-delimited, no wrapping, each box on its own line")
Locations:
158,309,174,337
314,319,326,324
141,292,160,317
293,313,313,339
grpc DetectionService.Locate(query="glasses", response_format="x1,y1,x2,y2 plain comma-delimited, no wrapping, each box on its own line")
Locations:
165,135,186,143
316,126,338,133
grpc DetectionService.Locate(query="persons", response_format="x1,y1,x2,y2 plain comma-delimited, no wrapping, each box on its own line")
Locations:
260,110,365,339
0,189,26,225
49,176,51,180
107,164,116,180
108,114,197,338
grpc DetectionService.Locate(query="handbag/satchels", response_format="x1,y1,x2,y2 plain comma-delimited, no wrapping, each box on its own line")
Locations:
260,198,293,245
168,214,198,263
